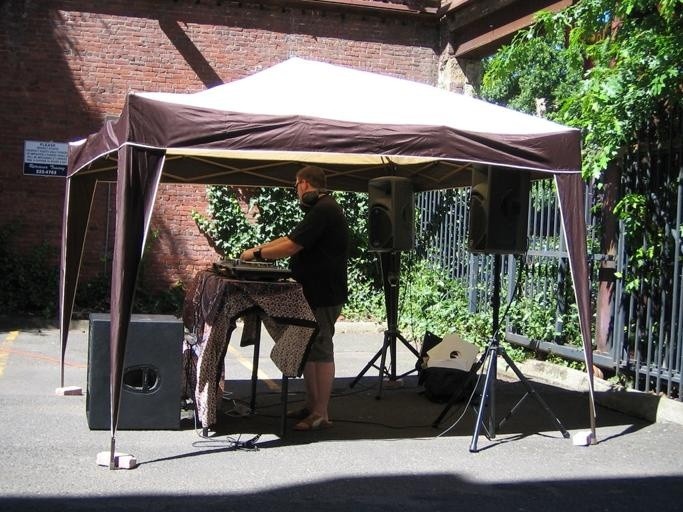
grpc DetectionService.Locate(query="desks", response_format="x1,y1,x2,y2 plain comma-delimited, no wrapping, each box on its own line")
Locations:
189,270,306,437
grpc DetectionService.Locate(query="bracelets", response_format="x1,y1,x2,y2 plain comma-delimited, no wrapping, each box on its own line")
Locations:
252,244,264,262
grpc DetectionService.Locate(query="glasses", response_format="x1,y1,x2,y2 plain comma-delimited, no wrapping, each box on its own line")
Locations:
294,181,301,191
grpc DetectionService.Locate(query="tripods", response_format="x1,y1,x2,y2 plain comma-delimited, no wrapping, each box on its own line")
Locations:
350,251,427,399
430,254,569,452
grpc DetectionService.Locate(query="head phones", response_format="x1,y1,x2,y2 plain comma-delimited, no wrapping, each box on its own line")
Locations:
300,188,333,215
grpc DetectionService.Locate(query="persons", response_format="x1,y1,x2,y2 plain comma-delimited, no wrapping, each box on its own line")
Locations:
235,163,352,429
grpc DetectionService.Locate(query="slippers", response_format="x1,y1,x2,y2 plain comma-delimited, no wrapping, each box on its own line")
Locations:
293,414,334,432
286,404,311,419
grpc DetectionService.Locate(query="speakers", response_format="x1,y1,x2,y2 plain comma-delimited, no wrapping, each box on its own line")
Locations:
367,174,417,252
468,164,530,254
86,314,185,431
